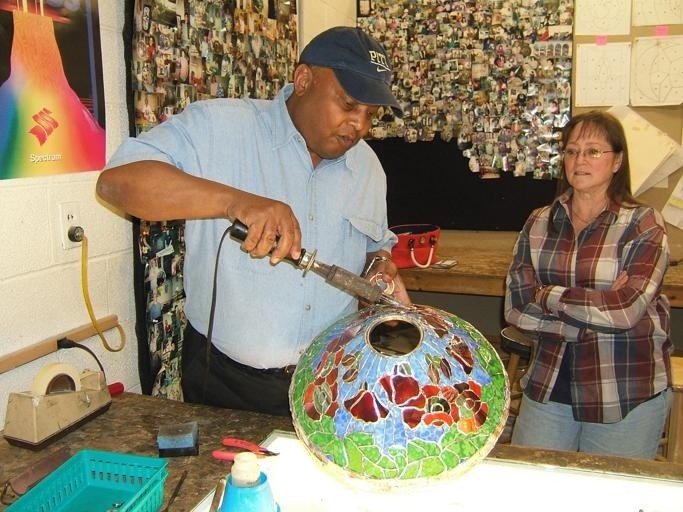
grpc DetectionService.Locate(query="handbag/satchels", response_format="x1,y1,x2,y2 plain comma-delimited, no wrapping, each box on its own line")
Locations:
387,224,440,269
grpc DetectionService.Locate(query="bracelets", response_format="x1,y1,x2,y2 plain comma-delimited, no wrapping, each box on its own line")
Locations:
361,253,391,278
531,284,548,302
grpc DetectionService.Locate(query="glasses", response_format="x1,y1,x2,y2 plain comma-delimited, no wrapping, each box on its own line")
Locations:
563,148,615,158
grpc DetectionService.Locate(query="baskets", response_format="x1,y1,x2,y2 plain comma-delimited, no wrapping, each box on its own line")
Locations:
2,449,169,512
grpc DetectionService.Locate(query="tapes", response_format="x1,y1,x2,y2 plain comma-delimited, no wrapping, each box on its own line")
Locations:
32,363,81,396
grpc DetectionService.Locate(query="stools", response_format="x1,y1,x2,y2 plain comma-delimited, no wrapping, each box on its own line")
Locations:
500,324,532,391
656,357,683,465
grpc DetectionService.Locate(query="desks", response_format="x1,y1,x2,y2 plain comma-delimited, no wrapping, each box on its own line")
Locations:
1,392,682,512
400,230,683,311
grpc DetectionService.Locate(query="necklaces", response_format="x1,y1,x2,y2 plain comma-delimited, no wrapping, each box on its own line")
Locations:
571,199,608,225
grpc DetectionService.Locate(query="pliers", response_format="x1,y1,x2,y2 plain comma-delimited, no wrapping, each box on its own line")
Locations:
212,438,280,461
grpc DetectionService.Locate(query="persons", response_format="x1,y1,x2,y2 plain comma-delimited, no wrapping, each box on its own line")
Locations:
95,23,413,421
504,112,672,461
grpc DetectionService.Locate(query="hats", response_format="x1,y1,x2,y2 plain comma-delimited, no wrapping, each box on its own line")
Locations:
299,26,403,119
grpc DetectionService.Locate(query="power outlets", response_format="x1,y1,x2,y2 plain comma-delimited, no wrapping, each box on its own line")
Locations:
60,202,86,250
